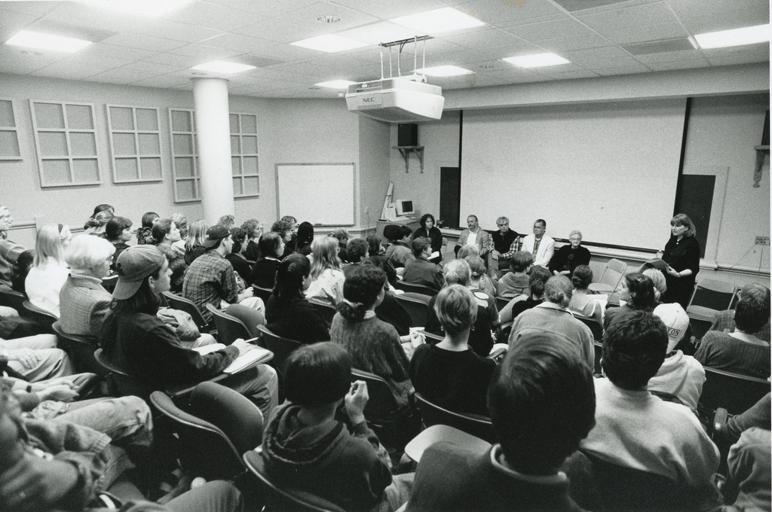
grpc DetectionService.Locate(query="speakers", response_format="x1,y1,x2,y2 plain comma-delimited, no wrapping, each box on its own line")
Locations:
762,110,770,145
398,124,417,146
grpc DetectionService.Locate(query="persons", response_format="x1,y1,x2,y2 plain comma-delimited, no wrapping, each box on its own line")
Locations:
1,204,772,511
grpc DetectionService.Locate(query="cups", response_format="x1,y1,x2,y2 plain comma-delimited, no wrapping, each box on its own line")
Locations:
410,325,426,349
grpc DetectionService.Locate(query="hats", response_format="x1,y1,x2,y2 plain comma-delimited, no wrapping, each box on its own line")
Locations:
201,225,231,248
112,245,165,301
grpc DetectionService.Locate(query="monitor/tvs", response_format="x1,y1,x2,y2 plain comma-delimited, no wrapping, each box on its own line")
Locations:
396,200,415,218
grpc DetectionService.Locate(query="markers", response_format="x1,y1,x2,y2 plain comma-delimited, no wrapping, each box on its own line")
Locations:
315,224,321,225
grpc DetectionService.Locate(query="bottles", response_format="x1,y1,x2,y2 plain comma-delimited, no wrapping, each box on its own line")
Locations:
656,250,664,259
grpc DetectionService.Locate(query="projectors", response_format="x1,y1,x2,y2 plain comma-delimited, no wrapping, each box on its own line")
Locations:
345,78,446,124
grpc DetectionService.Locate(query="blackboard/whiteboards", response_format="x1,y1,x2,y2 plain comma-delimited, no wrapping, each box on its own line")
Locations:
275,163,355,229
437,167,727,269
